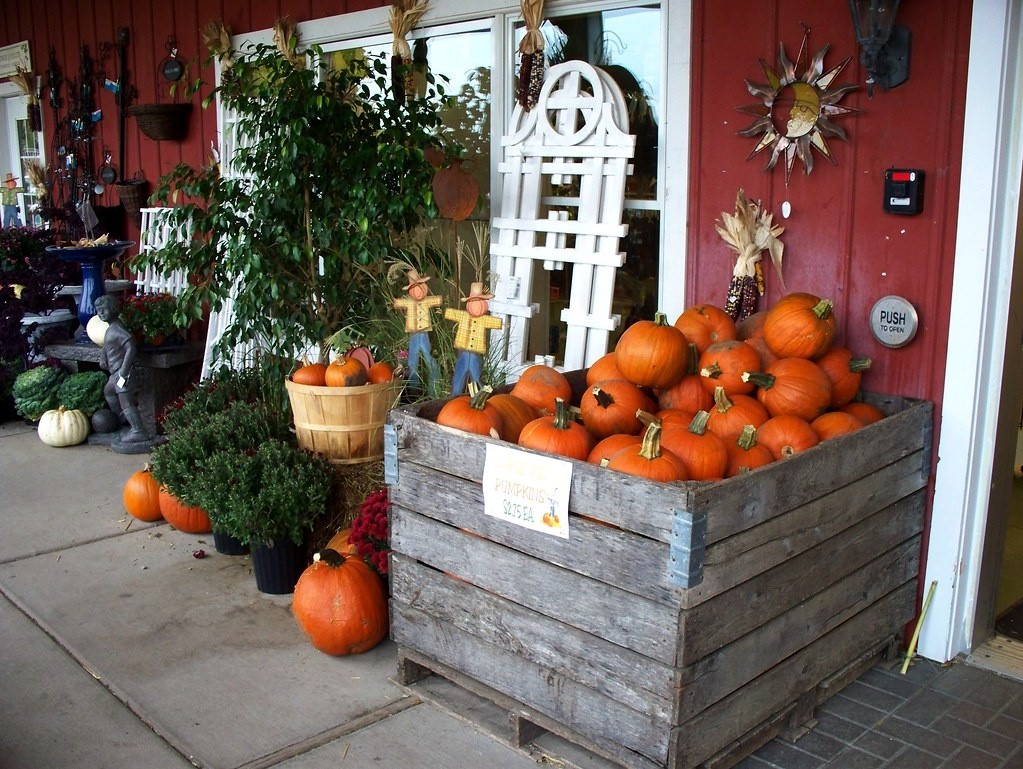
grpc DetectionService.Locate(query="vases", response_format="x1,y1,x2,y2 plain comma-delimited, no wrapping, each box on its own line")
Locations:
214,532,251,556
250,536,308,595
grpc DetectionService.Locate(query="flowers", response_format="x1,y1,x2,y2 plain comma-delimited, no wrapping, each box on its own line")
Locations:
144,350,343,548
118,291,180,344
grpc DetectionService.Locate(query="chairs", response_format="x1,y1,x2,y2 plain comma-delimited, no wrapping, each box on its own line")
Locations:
134,208,194,298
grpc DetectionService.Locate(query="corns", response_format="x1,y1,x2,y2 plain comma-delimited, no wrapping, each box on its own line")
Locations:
391,55,415,104
724,278,759,318
221,70,240,106
27,102,43,132
517,51,546,112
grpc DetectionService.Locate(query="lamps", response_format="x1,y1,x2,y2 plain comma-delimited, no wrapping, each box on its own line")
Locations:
849,0,913,100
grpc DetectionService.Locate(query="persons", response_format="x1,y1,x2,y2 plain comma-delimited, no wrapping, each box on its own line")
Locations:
95,295,150,443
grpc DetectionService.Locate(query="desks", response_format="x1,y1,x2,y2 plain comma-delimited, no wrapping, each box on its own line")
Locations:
54,279,134,338
6,309,77,364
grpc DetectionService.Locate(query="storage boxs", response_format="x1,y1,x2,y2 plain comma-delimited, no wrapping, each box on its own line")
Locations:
385,368,935,769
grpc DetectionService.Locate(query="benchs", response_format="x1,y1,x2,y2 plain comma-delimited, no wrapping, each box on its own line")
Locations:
44,342,204,435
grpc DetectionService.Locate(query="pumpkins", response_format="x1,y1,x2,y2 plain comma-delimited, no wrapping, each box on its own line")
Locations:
291,357,397,388
293,530,386,656
123,470,210,533
437,292,886,483
424,147,479,220
37,408,88,449
